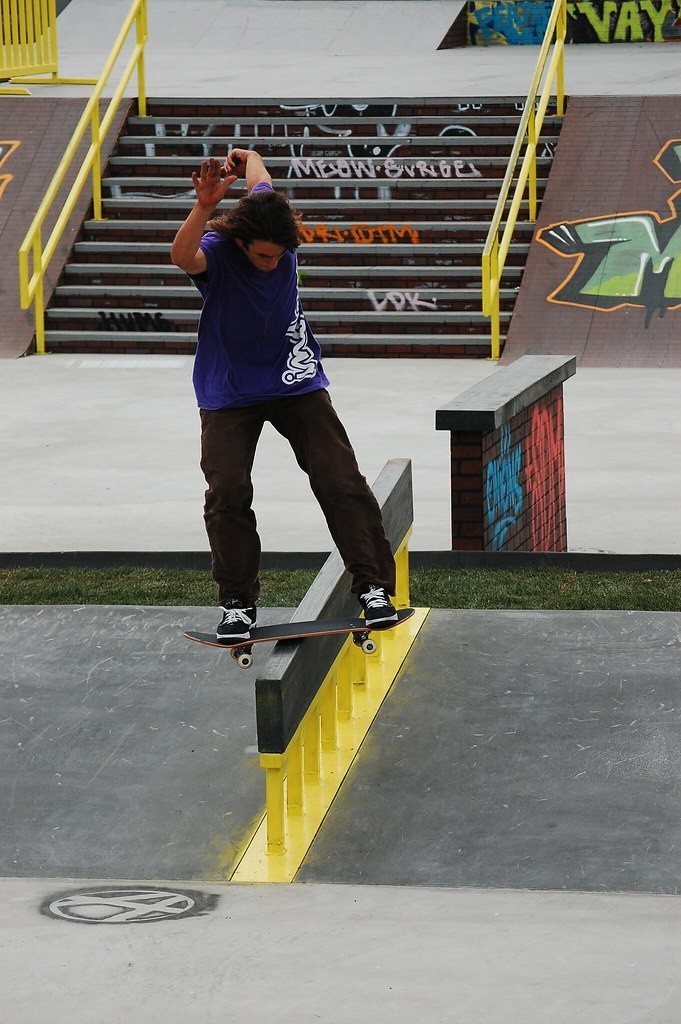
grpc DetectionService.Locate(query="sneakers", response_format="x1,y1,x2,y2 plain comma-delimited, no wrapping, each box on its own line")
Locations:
216,599,258,640
358,584,398,626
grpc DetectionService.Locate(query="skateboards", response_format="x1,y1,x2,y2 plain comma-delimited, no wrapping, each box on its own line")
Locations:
183,608,415,669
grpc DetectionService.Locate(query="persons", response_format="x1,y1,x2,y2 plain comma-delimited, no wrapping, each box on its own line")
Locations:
171,149,399,641
